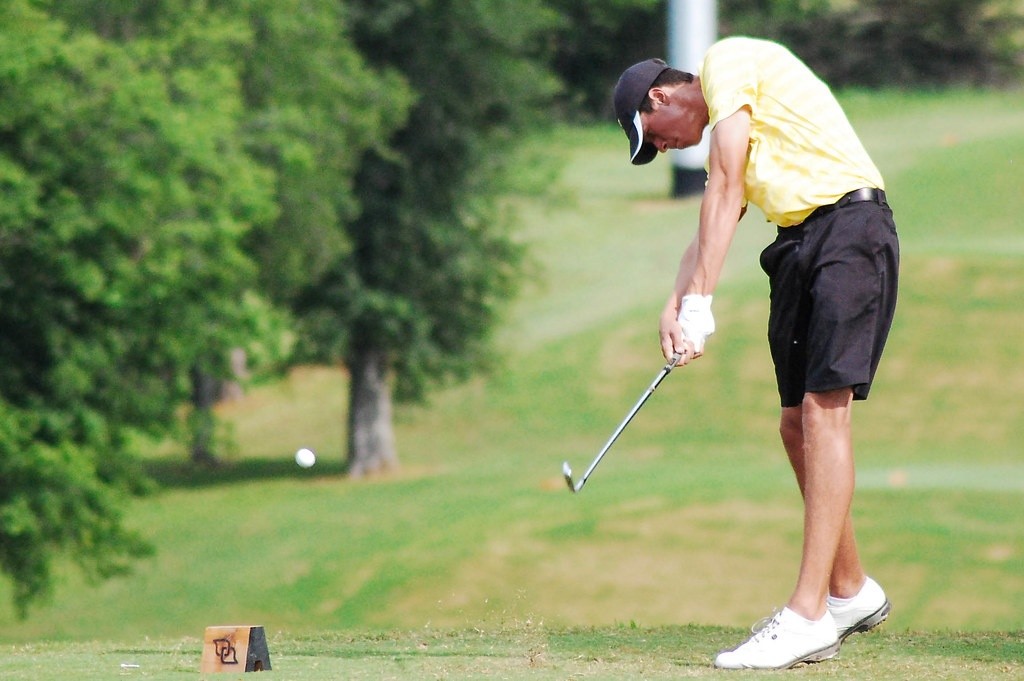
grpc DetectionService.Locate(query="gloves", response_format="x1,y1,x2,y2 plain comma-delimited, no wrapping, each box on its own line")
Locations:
677,295,716,353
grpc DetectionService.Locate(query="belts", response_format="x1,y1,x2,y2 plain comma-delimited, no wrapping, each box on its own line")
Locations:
778,189,889,232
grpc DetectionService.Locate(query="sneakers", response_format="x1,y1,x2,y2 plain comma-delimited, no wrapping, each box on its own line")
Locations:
714,609,839,669
827,575,890,645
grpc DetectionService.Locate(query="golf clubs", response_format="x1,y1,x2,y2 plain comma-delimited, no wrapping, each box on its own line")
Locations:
560,351,684,493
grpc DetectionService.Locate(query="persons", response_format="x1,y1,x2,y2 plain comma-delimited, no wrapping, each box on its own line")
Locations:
611,35,903,671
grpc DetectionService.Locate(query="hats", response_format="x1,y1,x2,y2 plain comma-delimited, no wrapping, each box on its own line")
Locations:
614,58,671,165
